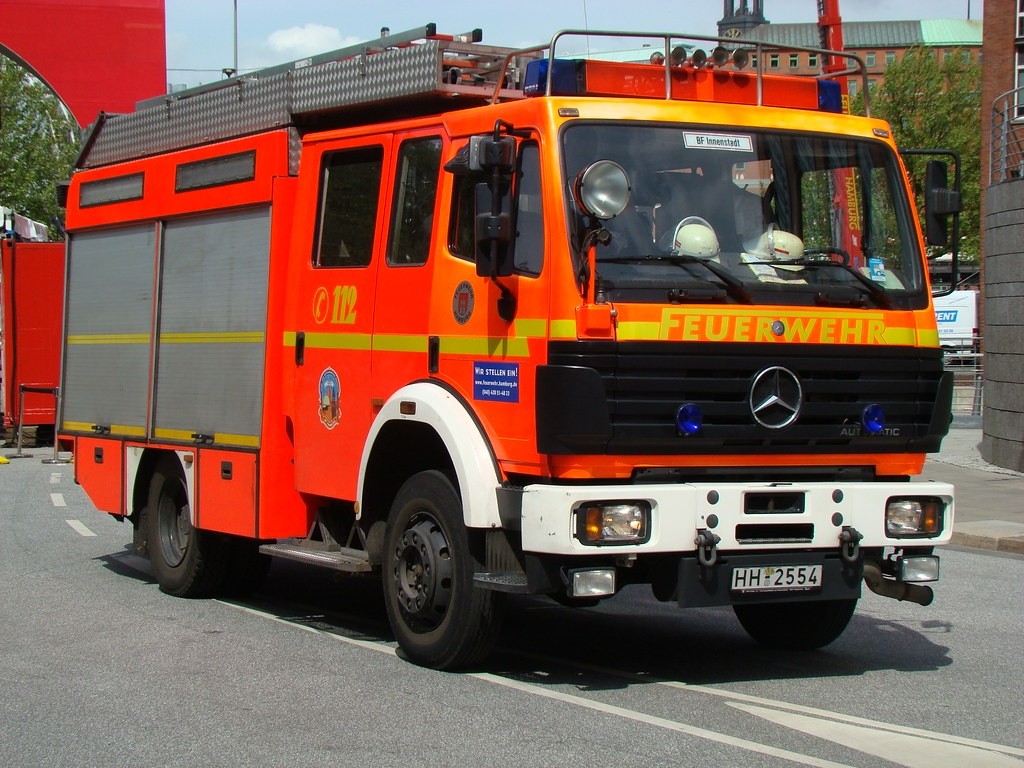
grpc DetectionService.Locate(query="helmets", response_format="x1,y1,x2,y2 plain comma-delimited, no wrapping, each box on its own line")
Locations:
673,222,719,258
749,230,804,261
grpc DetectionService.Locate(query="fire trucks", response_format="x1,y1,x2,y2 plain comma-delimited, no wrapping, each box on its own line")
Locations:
61,22,963,674
0,207,64,440
926,258,979,360
